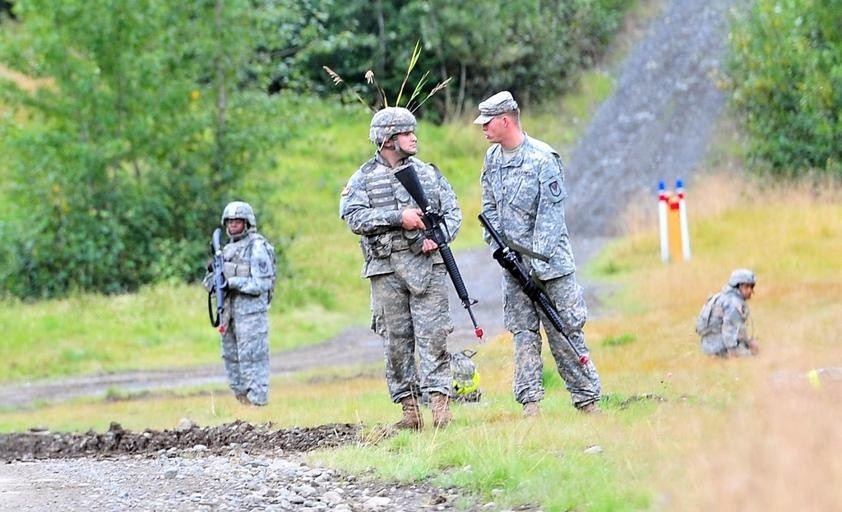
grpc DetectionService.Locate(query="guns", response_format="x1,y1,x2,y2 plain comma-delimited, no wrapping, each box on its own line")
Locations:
211,227,224,337
476,213,588,367
394,165,483,340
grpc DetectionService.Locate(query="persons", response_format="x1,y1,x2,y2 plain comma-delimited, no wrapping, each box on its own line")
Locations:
338,105,462,430
473,89,605,414
693,270,761,358
200,200,276,405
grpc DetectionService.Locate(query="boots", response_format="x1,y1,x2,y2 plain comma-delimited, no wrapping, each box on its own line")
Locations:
522,401,540,419
430,392,452,429
382,395,424,437
580,401,602,414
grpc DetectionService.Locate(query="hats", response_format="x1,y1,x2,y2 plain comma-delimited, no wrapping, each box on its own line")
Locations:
473,91,518,125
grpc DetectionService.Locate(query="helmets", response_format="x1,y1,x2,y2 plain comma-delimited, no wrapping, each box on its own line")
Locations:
369,107,417,144
220,201,257,238
728,269,755,287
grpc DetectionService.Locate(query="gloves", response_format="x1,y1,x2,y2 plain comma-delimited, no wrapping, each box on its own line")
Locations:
493,247,522,280
219,279,230,299
522,277,546,302
